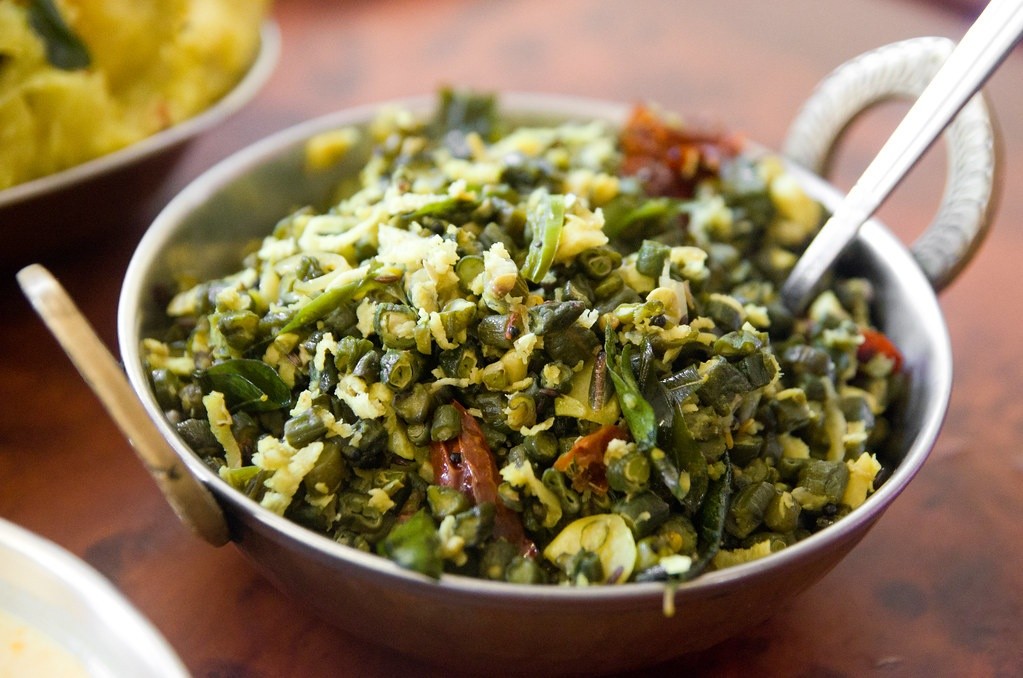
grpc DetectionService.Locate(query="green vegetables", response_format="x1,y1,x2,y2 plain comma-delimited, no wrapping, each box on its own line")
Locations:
20,0,94,71
150,79,909,587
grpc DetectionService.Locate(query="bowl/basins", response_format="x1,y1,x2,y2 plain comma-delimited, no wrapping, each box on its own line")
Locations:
0,8,283,218
0,526,199,678
12,39,1004,677
